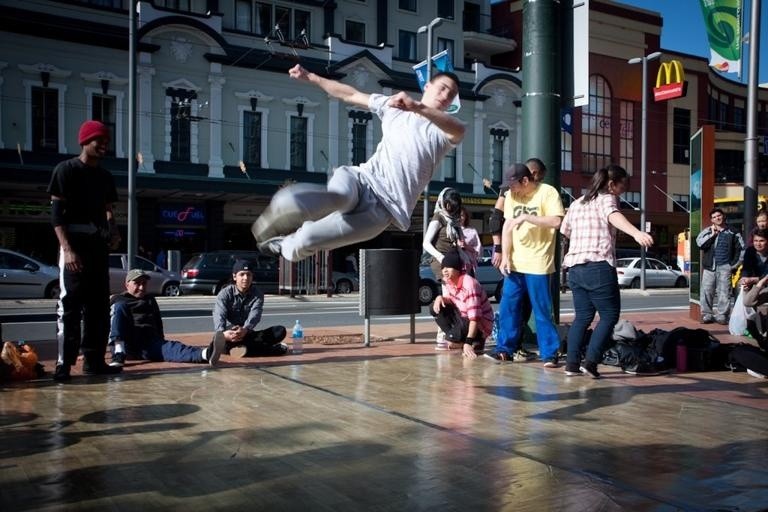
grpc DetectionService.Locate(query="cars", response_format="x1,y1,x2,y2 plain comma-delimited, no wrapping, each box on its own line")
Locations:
328,267,360,295
107,250,180,295
417,246,505,306
613,254,690,290
0,247,61,300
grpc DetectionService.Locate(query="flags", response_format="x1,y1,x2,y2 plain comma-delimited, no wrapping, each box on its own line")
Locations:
698,0,740,73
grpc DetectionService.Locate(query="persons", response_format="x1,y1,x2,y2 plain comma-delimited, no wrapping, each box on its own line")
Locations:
108,269,227,367
696,208,768,348
213,260,289,358
560,165,654,379
424,188,493,359
47,121,122,382
493,158,563,366
250,65,465,263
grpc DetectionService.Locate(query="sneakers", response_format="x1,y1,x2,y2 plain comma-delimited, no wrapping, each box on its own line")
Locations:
271,343,288,354
83,352,126,373
260,237,282,254
55,365,71,379
565,360,600,379
484,349,536,363
544,358,557,368
206,331,247,364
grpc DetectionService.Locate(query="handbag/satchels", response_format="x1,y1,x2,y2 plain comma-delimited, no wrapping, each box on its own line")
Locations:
601,319,768,377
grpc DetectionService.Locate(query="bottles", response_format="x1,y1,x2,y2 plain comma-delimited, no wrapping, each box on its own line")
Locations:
291,319,305,356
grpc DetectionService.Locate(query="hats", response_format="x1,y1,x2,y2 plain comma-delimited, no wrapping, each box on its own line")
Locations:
441,254,462,269
125,269,150,281
499,163,530,188
233,260,252,273
79,121,110,145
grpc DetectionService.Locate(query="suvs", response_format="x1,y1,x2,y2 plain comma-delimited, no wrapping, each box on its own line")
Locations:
180,246,293,294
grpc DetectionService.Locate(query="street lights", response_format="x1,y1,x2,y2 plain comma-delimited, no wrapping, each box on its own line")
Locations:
416,16,445,252
628,51,664,290
